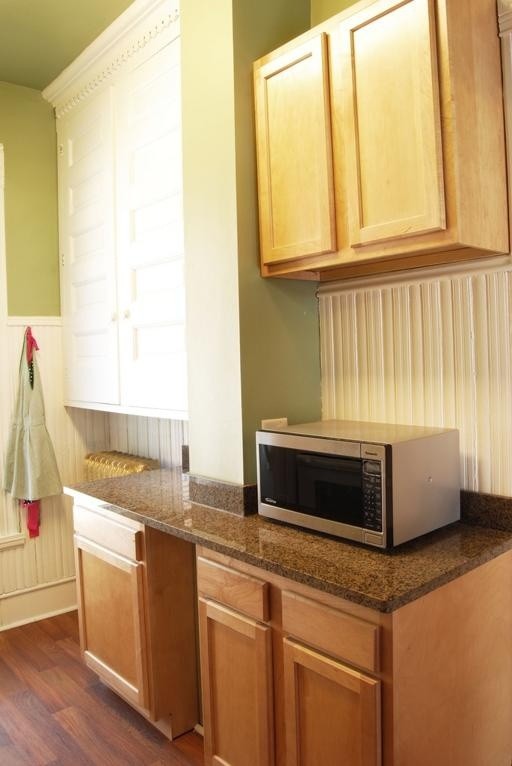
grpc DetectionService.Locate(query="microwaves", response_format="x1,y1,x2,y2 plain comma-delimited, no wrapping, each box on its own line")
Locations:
255,418,461,554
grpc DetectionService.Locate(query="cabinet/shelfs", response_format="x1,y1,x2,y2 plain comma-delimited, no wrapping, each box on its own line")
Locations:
193,544,512,765
73,497,203,740
254,2,511,281
60,34,186,418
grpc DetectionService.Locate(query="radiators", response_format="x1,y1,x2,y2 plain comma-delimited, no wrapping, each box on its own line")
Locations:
84,450,157,481
